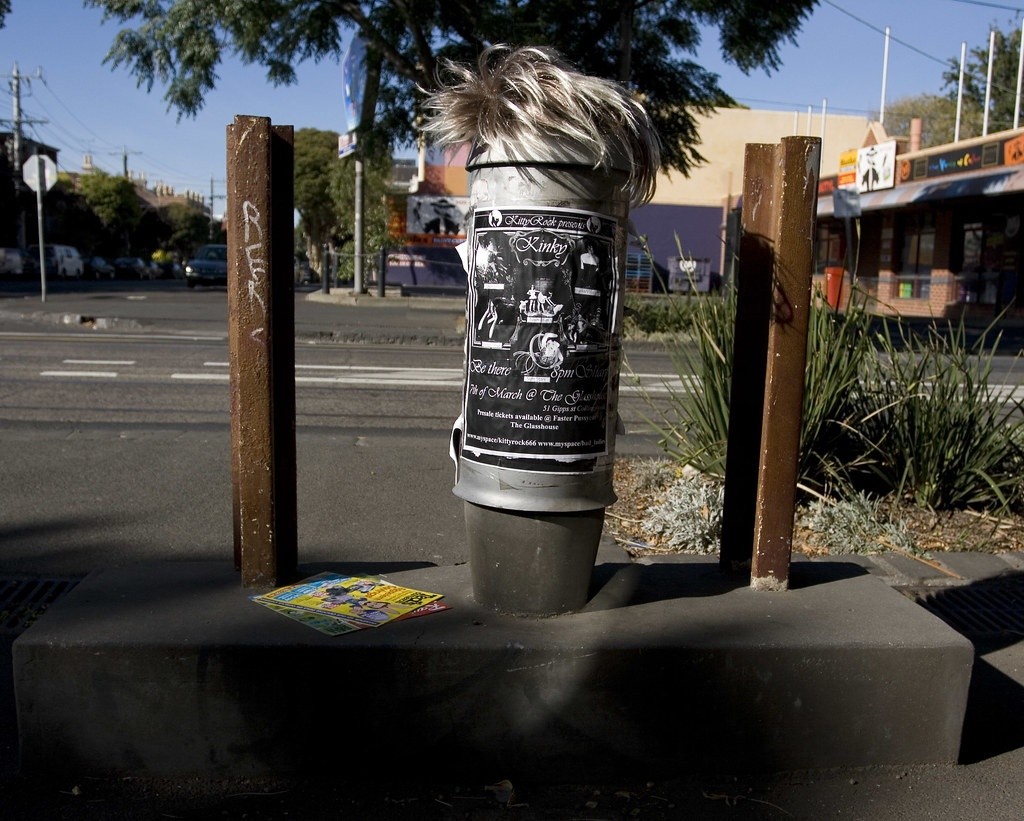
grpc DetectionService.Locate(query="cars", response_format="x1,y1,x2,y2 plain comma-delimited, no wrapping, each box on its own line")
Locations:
292,256,312,286
185,243,227,290
0,245,187,289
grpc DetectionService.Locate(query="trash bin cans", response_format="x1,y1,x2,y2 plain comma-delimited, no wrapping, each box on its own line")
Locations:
823,266,844,310
667,255,712,297
